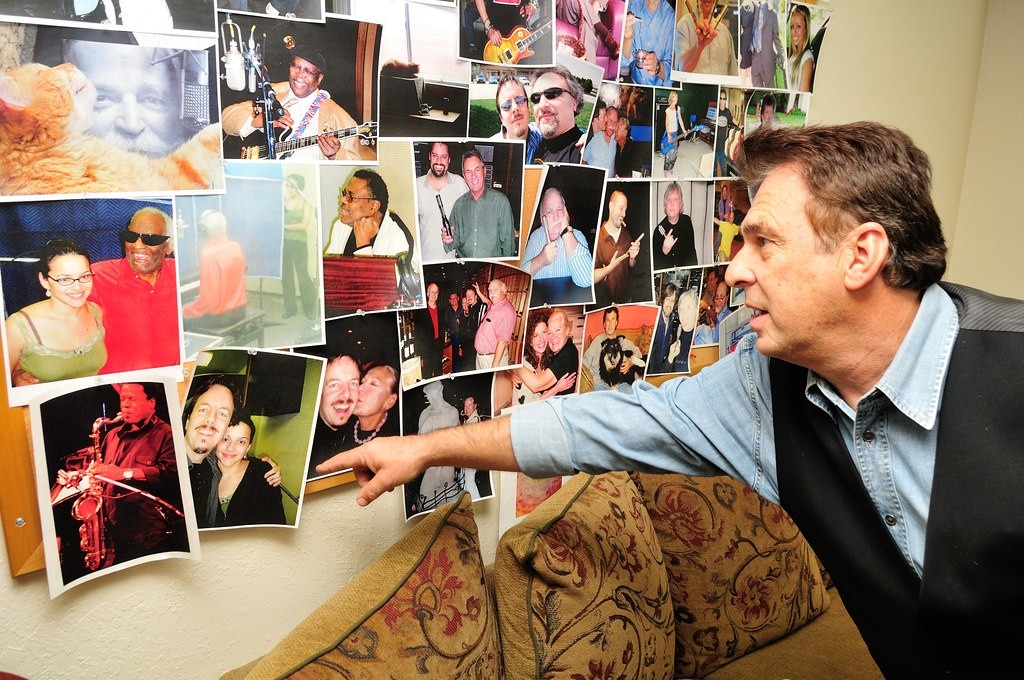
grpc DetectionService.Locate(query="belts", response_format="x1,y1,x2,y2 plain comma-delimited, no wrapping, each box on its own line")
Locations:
478,352,496,355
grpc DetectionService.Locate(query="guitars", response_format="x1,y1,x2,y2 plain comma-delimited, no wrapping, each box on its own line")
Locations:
659,124,706,155
222,120,377,163
482,18,553,66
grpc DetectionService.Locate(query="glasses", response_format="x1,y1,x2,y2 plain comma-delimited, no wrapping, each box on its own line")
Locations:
121,231,171,246
341,190,377,203
530,87,576,104
500,96,528,111
720,97,727,101
714,294,727,302
287,61,321,79
46,270,95,286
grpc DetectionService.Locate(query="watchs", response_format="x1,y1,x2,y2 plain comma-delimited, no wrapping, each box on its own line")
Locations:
123,468,133,480
656,59,661,75
561,225,573,238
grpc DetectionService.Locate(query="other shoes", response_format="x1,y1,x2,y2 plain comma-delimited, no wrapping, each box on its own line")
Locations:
283,312,295,318
308,314,314,320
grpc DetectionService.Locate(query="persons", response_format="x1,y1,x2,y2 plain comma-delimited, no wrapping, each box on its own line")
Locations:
317,123,1024,680
0,0,815,574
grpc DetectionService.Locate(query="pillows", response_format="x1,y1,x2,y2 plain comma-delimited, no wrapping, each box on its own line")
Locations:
622,470,831,680
493,469,676,680
239,486,507,680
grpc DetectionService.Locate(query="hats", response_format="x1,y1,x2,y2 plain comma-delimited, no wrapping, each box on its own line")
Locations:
294,47,327,72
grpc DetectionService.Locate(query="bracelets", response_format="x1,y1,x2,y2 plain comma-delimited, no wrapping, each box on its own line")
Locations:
484,18,494,35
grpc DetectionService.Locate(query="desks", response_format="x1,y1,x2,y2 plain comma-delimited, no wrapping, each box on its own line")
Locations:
380,108,461,127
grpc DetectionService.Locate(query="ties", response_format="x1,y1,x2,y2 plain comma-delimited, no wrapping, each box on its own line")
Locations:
758,5,764,52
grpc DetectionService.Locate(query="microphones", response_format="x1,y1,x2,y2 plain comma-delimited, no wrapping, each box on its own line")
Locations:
249,35,257,94
225,39,245,91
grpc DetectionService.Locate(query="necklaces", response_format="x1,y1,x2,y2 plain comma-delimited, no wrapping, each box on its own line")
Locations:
354,412,388,444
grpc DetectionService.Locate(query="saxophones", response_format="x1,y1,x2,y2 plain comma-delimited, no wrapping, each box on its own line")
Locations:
71,411,127,573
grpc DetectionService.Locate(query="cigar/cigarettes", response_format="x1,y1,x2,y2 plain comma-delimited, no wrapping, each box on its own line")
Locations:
621,219,626,226
